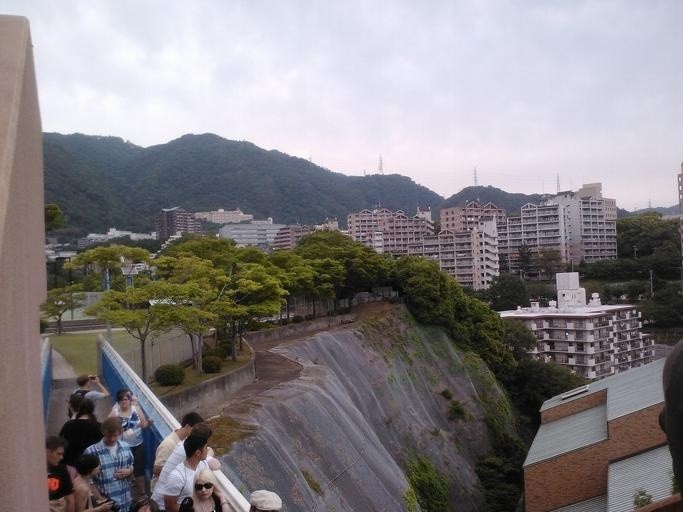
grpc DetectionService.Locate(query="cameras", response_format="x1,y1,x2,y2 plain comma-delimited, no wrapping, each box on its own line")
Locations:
89,376,96,380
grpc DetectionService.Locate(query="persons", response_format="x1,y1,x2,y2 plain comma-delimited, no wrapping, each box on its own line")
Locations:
46,375,283,512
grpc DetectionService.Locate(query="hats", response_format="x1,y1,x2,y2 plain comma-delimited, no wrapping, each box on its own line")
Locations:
250,490,283,510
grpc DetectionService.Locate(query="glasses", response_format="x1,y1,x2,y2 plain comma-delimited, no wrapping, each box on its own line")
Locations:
194,482,213,490
